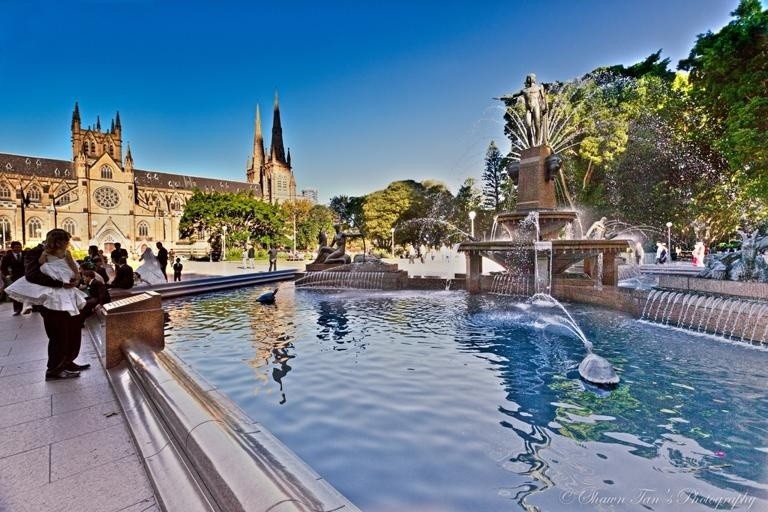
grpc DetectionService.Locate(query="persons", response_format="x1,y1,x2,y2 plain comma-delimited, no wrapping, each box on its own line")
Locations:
503,72,547,148
248,245,255,269
268,245,276,271
654,240,711,268
636,242,645,265
0,227,183,382
313,223,363,264
287,250,319,262
242,248,248,269
398,242,450,265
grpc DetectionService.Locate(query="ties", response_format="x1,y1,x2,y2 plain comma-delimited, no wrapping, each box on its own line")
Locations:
17,254,20,259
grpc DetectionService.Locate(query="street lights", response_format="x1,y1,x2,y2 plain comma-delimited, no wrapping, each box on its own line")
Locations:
468,211,477,240
665,222,673,263
223,225,227,262
391,229,395,258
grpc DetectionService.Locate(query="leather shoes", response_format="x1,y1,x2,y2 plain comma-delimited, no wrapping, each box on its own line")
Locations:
67,362,90,371
46,369,80,380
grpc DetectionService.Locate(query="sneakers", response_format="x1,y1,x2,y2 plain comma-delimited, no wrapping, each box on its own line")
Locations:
24,305,32,315
12,311,20,316
